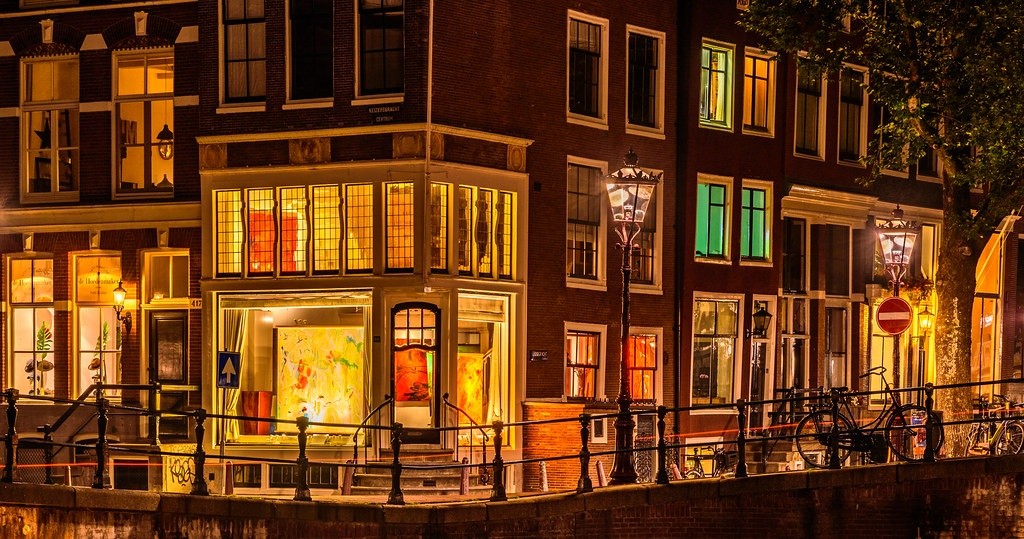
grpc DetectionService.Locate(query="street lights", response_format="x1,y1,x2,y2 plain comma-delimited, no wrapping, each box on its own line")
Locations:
875,204,924,457
603,145,664,485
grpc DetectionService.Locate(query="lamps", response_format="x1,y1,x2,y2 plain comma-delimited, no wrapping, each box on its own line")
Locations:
752,305,773,335
109,280,132,329
155,123,173,160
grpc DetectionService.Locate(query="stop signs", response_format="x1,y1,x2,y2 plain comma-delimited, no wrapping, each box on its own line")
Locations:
876,297,912,336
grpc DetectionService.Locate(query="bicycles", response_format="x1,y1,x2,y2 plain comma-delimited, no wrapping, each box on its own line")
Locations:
795,365,945,469
965,393,1024,455
679,445,739,478
